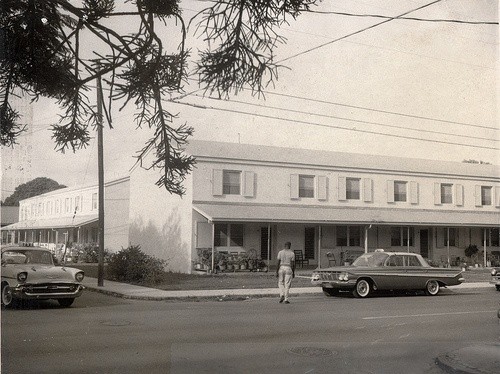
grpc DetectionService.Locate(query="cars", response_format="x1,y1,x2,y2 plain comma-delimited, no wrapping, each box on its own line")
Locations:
0,245,87,309
312,248,466,298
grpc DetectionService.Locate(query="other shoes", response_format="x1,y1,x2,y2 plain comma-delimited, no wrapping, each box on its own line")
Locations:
279,296,283,303
285,301,290,304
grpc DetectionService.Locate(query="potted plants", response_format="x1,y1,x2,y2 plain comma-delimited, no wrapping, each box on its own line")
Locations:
194,248,267,273
70,243,99,263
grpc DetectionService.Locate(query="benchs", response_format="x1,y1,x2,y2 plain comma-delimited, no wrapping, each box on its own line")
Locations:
340,250,364,266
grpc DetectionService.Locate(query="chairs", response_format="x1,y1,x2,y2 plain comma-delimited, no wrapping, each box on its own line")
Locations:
326,252,337,268
293,249,309,269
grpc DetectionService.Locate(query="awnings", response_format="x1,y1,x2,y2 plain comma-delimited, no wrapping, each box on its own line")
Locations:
192,198,500,277
0,216,102,247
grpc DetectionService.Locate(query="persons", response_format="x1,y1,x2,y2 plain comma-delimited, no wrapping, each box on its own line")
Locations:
271,241,299,305
